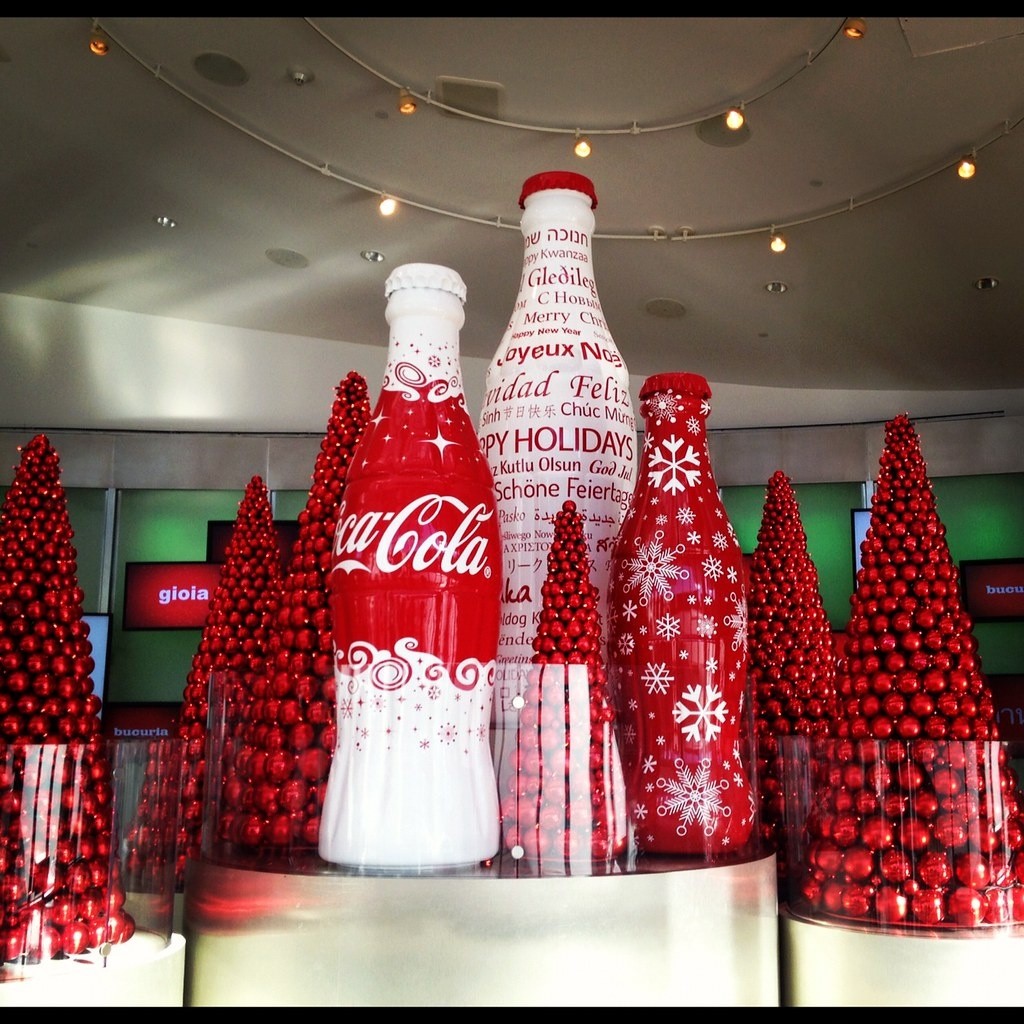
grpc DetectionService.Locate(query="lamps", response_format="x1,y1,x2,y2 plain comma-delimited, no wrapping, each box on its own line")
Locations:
398,88,417,115
574,135,593,157
958,156,976,178
89,31,111,56
726,106,747,129
844,18,867,39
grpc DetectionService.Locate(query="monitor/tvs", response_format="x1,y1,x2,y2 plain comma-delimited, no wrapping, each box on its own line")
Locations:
103,700,183,741
959,557,1024,623
122,561,227,631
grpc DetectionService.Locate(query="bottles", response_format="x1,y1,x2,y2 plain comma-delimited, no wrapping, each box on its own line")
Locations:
608,374,756,853
478,171,636,862
317,263,501,874
